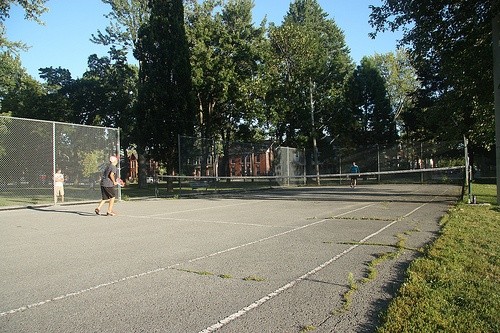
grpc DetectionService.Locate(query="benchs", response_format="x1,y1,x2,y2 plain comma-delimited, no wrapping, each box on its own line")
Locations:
189,180,210,192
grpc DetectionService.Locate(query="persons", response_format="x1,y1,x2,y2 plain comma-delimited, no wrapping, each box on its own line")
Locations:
52,170,65,203
349,161,360,190
94,156,119,215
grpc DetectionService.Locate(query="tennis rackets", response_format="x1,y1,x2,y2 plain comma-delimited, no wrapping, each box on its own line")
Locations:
116,177,125,187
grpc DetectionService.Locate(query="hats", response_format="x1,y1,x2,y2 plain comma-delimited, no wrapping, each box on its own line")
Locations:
110,156,117,162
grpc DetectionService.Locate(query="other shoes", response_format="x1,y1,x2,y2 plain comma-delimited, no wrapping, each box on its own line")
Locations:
95,207,101,214
107,212,115,216
